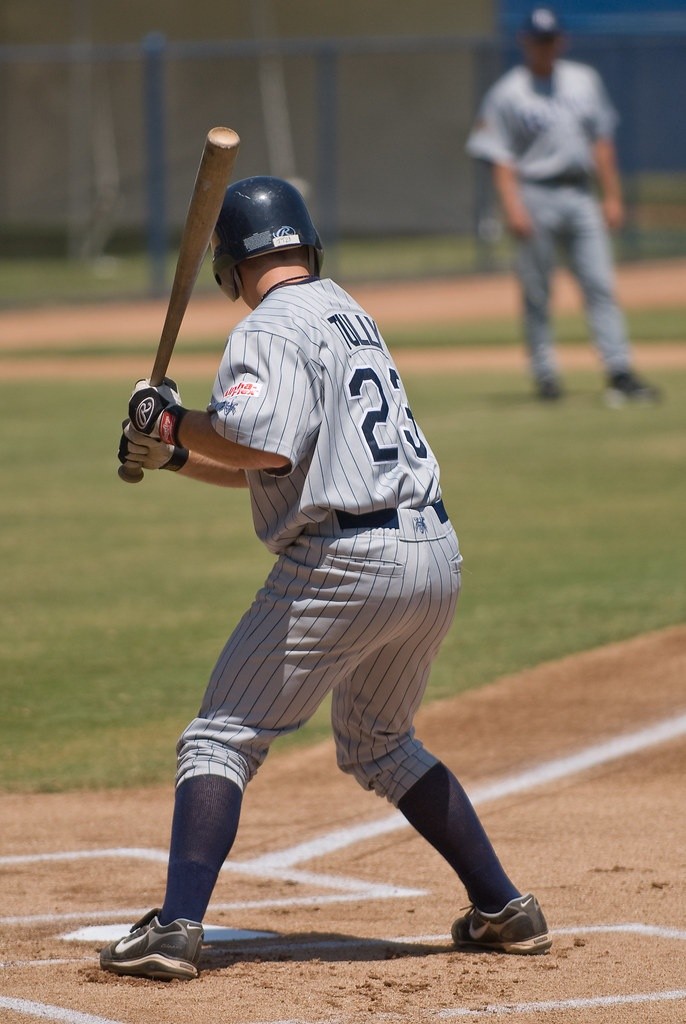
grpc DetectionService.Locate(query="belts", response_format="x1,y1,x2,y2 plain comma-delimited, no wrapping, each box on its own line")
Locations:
334,499,451,529
537,173,589,187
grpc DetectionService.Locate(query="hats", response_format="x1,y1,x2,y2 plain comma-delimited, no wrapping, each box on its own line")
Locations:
524,6,567,40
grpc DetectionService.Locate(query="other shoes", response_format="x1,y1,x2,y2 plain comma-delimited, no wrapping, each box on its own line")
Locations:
607,372,649,395
535,376,562,399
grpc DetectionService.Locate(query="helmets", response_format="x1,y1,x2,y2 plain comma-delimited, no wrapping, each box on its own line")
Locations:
207,175,325,302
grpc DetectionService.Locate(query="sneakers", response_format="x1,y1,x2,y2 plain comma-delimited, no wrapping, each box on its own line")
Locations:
99,907,205,980
450,892,554,954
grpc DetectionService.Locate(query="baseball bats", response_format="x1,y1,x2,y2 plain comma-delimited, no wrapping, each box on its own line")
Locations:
116,124,241,484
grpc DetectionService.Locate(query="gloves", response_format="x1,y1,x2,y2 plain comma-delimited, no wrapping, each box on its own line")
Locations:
128,376,191,450
117,417,189,472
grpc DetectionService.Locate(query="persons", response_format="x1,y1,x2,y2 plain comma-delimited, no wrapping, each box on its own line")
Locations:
466,8,659,403
103,176,554,980
